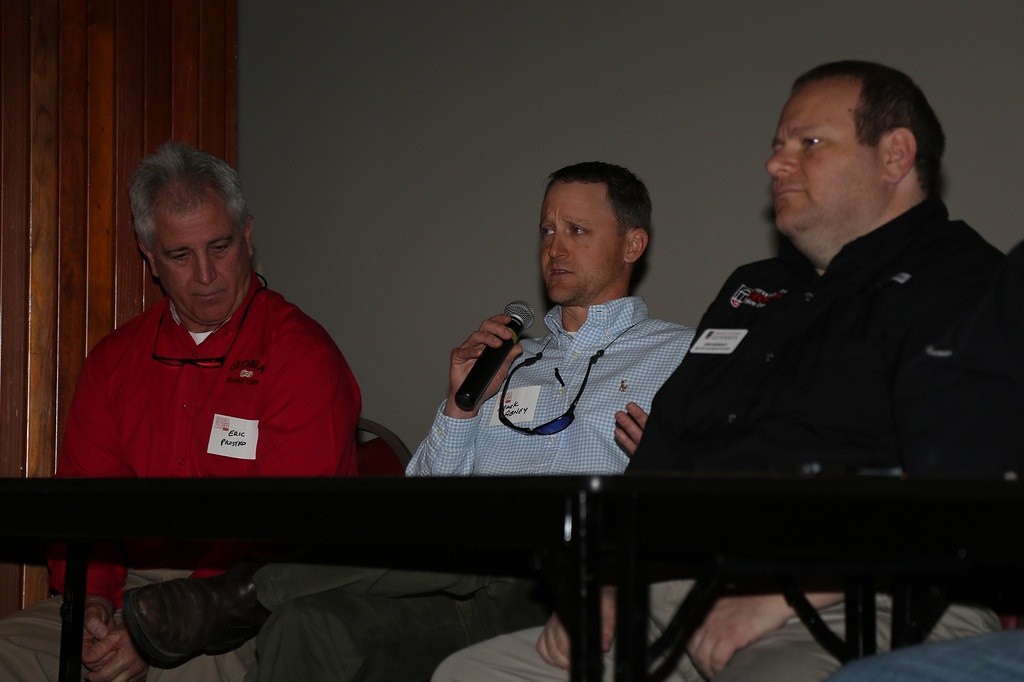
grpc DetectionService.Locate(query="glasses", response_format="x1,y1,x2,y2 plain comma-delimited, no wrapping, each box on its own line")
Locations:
151,302,251,369
497,350,604,436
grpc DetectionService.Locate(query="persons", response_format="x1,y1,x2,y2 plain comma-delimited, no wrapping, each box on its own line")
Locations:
0,61,1024,682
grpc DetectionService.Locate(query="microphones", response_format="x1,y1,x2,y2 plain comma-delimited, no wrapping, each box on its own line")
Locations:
454,301,535,412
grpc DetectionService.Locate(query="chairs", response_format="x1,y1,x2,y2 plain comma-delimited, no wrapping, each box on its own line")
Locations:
350,417,412,474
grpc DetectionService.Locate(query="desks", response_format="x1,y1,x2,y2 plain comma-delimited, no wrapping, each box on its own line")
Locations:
0,470,1024,682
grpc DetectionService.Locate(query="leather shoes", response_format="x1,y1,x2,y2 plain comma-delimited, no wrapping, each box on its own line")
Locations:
122,566,272,669
